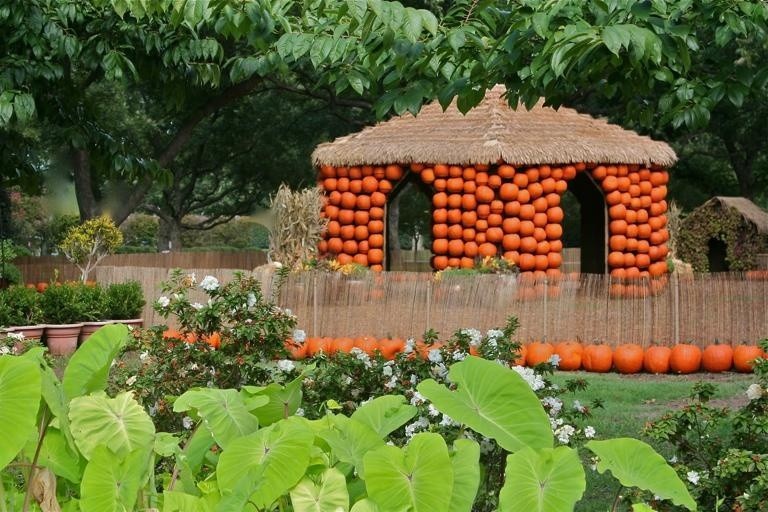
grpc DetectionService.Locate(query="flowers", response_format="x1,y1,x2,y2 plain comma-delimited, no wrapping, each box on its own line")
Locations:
435,254,516,281
290,258,373,280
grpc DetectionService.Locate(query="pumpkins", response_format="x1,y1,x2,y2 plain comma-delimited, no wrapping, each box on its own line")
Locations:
312,159,671,299
162,329,768,374
26,282,62,292
745,269,768,281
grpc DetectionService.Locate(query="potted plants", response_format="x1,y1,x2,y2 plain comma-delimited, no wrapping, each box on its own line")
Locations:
0,285,47,359
75,282,112,349
105,277,146,350
40,279,83,355
0,304,13,356
